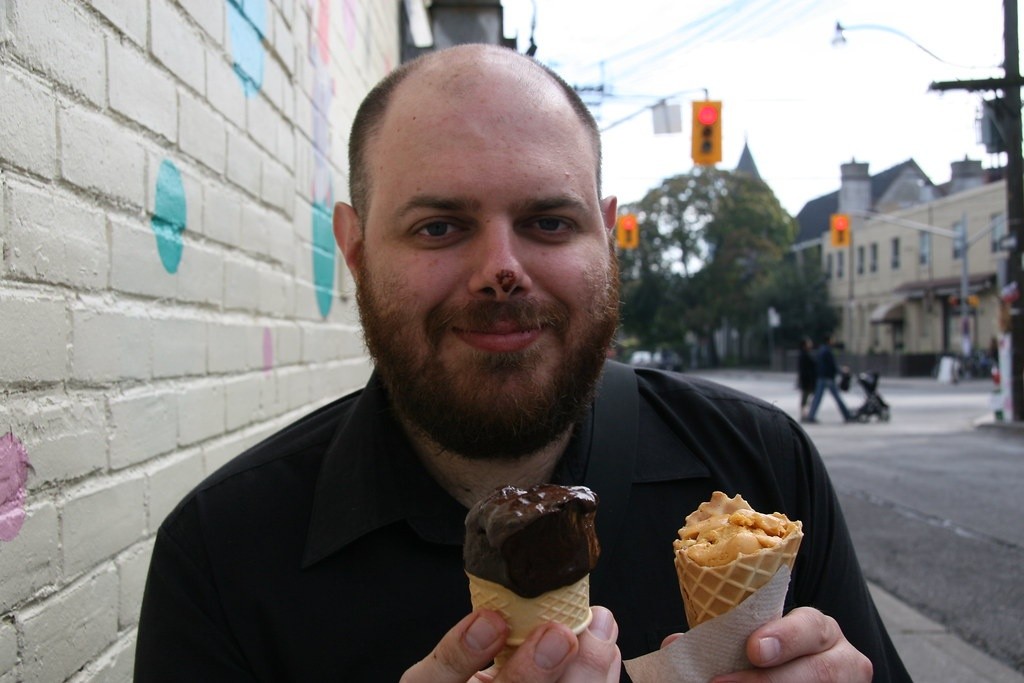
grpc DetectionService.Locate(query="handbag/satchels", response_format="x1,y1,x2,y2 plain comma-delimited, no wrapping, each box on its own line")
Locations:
840,372,851,390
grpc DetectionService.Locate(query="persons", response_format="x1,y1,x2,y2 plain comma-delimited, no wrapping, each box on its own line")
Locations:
132,42,911,683
796,331,854,424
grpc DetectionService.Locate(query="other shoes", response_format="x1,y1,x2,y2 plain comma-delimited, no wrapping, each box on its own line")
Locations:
801,416,819,424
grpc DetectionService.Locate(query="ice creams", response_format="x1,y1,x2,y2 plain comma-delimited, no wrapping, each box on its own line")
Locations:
673,491,804,630
461,481,600,674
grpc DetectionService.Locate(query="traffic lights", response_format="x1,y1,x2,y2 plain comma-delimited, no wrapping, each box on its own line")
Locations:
691,100,723,166
617,214,638,249
830,213,850,246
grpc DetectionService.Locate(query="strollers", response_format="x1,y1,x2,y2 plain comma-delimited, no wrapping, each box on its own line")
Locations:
846,367,890,424
